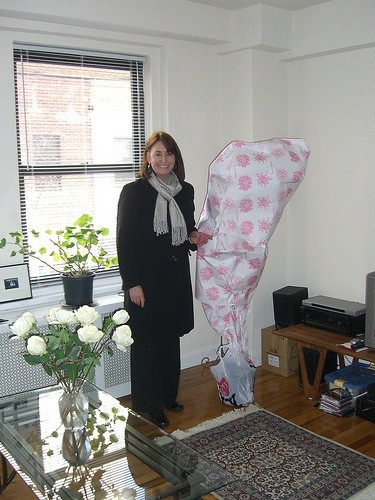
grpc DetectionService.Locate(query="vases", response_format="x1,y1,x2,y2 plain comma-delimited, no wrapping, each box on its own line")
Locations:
58,390,89,431
62,429,91,467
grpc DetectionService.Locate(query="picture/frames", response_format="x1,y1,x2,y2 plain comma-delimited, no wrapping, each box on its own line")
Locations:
0,263,33,304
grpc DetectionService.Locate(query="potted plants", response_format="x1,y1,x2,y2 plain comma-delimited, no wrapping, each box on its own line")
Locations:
0,214,118,307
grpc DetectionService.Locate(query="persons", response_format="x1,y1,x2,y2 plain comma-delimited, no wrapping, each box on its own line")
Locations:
116,132,212,429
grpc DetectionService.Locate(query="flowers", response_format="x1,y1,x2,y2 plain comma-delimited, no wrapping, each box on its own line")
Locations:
39,465,136,500
9,305,134,429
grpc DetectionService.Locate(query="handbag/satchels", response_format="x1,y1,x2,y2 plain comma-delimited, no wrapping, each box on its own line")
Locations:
209,343,256,407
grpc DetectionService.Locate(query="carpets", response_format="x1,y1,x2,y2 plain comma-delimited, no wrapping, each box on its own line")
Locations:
146,403,375,500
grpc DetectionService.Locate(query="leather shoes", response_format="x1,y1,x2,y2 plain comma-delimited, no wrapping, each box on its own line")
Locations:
147,407,169,427
161,399,184,410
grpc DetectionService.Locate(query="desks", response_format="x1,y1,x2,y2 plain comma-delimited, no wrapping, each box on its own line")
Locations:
272,324,375,399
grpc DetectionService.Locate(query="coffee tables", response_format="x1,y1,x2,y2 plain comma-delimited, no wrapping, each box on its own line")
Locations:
0,377,241,500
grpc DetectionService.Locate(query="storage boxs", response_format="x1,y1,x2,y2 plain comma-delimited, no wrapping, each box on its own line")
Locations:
262,325,298,377
325,362,375,408
